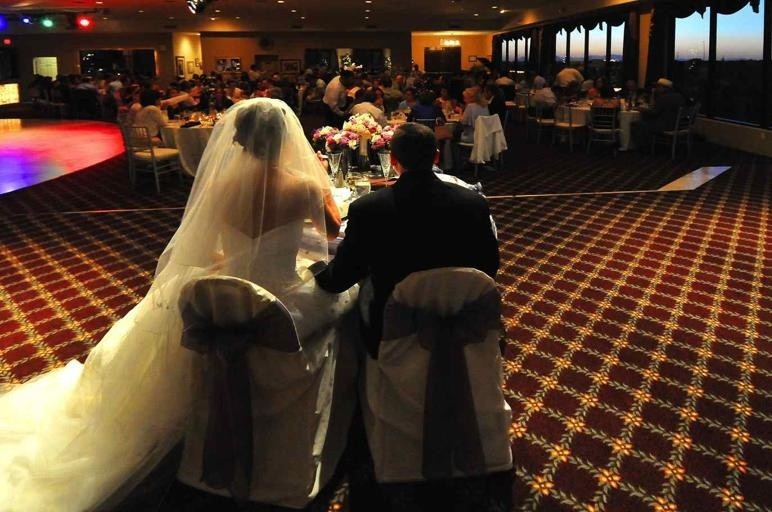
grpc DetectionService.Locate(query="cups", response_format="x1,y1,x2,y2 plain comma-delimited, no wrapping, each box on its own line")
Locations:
369,165,382,175
391,112,395,121
176,114,190,126
355,172,371,199
436,117,441,127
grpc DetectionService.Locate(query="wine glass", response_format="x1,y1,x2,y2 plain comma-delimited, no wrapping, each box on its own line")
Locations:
378,151,392,191
347,172,362,198
327,150,341,181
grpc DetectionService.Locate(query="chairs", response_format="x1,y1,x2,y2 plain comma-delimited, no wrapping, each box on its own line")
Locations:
355,265,513,482
30,75,697,221
174,275,368,506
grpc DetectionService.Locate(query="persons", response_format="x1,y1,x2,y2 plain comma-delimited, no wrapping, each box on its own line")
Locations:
306,121,501,361
295,59,504,180
172,97,360,342
490,58,688,157
169,63,295,118
29,68,168,149
